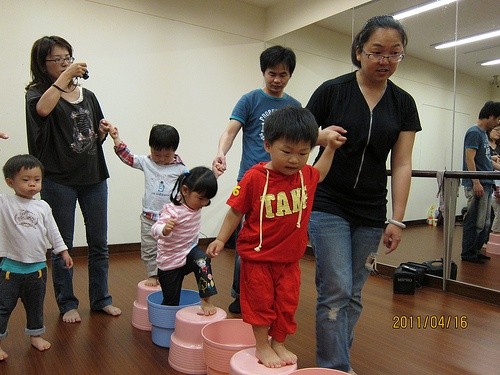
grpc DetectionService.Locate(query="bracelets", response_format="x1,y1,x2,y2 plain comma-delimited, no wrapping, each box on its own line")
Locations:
388,219,405,229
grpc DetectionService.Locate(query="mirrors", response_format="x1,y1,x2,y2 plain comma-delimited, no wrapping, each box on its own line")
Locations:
263,0,500,306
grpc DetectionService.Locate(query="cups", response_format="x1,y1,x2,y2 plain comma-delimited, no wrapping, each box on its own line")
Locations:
433,219,438,227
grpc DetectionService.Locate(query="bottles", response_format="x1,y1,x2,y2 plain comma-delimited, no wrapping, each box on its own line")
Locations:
428,205,436,226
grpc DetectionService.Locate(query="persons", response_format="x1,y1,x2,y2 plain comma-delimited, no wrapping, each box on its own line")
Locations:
304,16,428,373
214,44,307,316
101,120,226,288
24,36,125,323
205,105,346,368
148,159,224,314
460,99,500,264
0,154,76,361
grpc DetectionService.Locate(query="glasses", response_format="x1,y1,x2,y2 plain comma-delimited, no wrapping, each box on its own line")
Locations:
359,45,405,64
43,56,75,64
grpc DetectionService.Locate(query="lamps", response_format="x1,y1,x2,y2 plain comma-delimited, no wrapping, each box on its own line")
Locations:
390,0,500,66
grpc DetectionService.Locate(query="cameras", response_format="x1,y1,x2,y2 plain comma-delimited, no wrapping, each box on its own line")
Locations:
80,71,89,80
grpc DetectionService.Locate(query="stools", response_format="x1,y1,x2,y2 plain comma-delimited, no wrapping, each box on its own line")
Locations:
132,279,162,331
485,232,500,255
230,348,297,375
167,306,227,375
